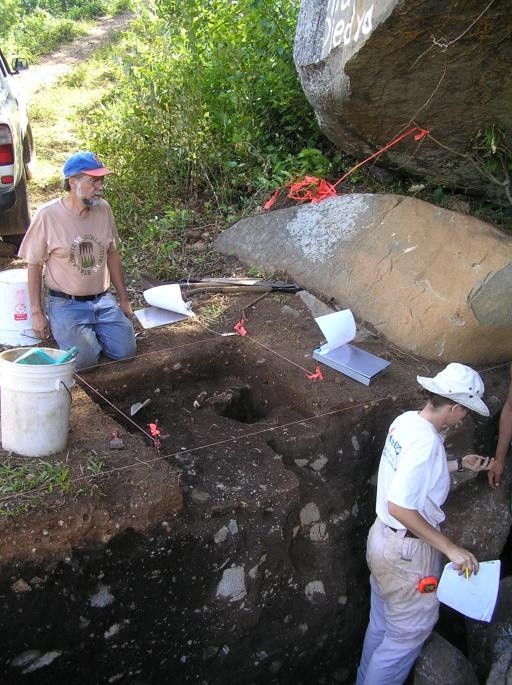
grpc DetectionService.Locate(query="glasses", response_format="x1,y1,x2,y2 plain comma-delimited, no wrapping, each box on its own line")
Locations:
75,175,106,187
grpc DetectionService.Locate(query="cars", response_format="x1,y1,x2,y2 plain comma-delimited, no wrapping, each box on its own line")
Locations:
1,49,35,228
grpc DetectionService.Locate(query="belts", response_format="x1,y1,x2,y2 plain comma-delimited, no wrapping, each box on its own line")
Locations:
387,525,422,539
50,289,110,304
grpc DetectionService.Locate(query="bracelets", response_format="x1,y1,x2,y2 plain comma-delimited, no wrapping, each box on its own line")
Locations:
455,456,463,473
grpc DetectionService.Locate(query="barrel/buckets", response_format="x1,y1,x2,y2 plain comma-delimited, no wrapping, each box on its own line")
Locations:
0,268,46,346
0,346,75,458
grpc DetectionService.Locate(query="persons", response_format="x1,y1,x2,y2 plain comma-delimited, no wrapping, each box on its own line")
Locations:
487,382,511,492
17,148,135,376
352,363,492,684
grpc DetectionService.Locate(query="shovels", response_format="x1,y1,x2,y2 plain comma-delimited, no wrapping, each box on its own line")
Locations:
16,346,81,364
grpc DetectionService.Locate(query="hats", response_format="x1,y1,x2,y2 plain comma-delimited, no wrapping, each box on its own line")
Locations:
413,361,493,420
62,149,117,178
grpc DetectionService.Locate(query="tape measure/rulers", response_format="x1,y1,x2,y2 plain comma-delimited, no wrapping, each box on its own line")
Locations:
418,578,438,593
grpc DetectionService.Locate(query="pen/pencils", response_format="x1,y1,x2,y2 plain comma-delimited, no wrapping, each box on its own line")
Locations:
467,567,469,581
